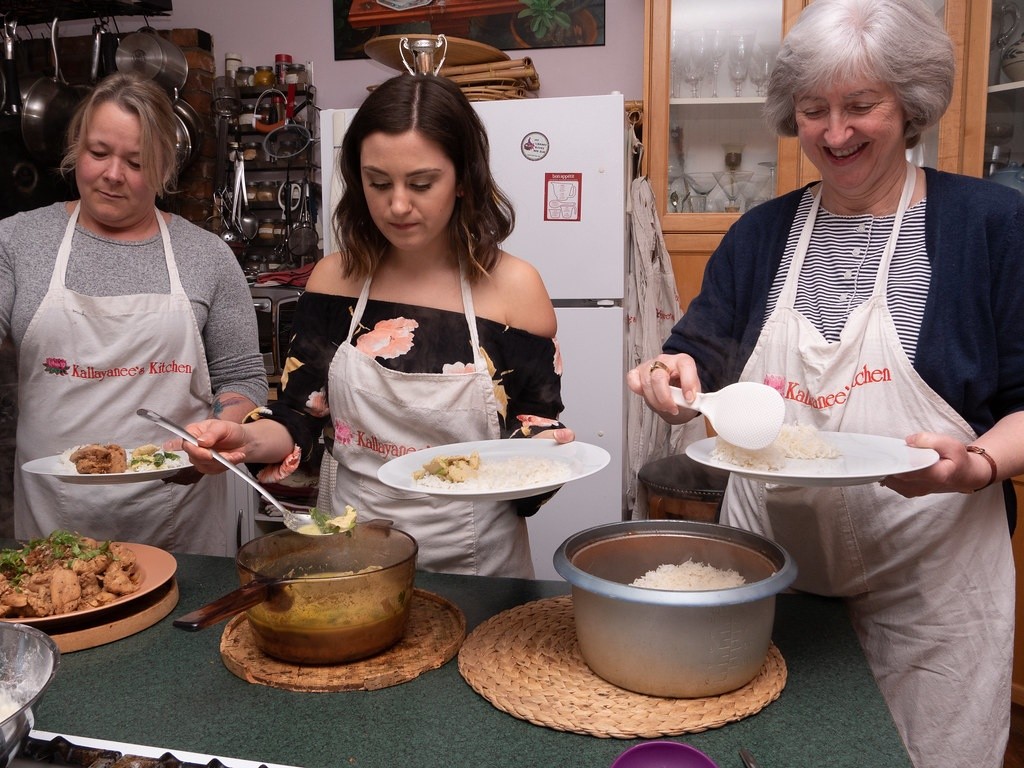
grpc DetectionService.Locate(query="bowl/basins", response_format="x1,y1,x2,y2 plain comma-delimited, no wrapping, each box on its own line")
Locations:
0,620,60,760
609,740,719,768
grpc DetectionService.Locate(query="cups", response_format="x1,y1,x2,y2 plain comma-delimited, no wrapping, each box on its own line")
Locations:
274,54,292,84
224,53,242,87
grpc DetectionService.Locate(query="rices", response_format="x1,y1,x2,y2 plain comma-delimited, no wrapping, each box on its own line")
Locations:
627,559,749,590
419,456,566,490
710,431,788,473
774,421,843,459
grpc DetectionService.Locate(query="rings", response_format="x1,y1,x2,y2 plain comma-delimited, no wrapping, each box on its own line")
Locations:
649,361,672,376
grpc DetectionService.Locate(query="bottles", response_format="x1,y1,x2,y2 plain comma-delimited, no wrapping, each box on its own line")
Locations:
218,52,321,281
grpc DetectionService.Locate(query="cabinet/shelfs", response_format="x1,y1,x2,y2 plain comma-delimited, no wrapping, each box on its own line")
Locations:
217,83,316,274
645,0,1024,320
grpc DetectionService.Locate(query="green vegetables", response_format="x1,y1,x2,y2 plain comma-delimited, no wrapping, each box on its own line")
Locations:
310,506,341,534
0,530,121,594
131,452,180,467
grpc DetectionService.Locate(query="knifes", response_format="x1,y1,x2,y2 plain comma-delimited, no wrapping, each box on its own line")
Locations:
739,748,761,768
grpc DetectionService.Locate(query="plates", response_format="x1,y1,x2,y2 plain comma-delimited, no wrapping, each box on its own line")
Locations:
19,447,195,485
376,438,610,501
685,430,940,486
0,541,178,623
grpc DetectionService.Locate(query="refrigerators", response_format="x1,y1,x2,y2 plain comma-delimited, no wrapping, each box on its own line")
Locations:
321,93,662,580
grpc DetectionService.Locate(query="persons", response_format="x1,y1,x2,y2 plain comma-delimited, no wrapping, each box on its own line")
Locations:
0,73,269,558
182,74,574,580
626,0,1024,768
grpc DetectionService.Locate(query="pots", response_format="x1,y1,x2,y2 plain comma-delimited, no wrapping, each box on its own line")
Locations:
552,519,800,700
172,518,419,667
0,18,209,218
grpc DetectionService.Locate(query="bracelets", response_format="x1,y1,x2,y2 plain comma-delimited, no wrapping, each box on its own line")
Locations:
958,445,998,496
